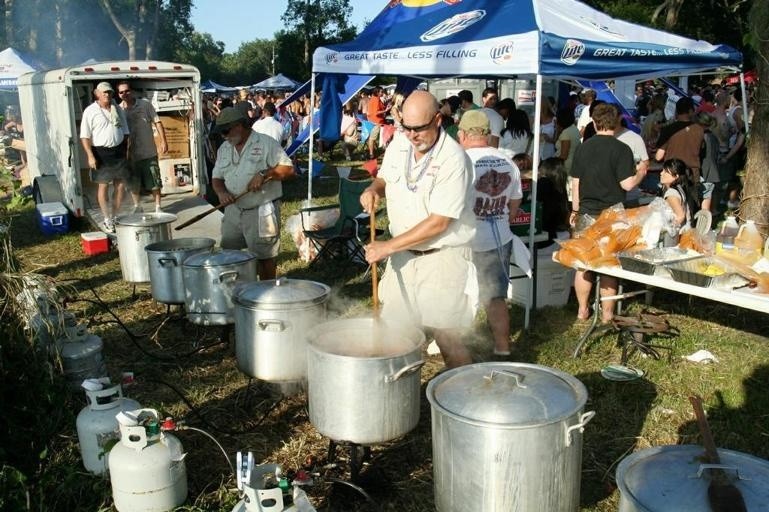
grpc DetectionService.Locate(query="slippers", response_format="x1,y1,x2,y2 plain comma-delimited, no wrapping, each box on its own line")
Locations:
576,307,625,327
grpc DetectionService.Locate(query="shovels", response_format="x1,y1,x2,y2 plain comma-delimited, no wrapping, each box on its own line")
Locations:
688,393,748,512
174,176,273,230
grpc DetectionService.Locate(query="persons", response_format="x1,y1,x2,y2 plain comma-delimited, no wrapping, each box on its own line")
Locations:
4,103,27,179
201,88,402,161
455,109,524,361
442,87,756,224
79,82,129,234
361,90,480,369
117,83,168,214
211,109,295,281
570,105,646,328
658,158,693,247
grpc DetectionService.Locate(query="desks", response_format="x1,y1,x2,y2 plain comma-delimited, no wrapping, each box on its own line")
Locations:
552,252,769,359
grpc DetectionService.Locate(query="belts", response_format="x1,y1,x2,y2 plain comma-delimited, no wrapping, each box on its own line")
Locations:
237,207,254,211
409,248,440,256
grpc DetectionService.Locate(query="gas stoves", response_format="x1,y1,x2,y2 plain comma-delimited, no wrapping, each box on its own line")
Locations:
328,436,416,483
165,302,185,317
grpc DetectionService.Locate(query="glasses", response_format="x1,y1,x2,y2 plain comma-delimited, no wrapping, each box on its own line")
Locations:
220,123,241,136
400,111,441,132
655,118,665,124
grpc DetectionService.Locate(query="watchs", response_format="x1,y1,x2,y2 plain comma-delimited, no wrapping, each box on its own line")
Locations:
258,169,268,179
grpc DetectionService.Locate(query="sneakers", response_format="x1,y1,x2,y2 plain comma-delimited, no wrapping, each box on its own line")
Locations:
103,215,121,232
132,207,166,213
369,155,379,162
338,155,355,161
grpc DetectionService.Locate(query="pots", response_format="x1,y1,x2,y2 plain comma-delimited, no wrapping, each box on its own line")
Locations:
181,245,258,326
425,359,596,512
303,316,427,445
614,444,769,512
145,237,216,303
113,211,178,283
231,276,332,382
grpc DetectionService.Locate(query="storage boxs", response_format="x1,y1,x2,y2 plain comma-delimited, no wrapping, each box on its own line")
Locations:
81,231,109,256
151,116,190,160
37,201,69,239
157,158,194,195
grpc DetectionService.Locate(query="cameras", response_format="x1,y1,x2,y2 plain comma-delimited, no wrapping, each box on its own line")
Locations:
634,157,641,165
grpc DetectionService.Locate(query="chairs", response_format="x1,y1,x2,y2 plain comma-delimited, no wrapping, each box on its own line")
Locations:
645,209,712,304
300,177,384,278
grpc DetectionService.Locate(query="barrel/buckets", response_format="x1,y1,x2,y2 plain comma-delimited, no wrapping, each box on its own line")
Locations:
716,216,739,257
734,220,762,265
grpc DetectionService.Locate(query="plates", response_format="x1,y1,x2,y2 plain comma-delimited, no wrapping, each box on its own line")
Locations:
600,365,644,382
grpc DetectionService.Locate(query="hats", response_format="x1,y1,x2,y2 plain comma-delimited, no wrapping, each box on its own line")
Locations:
213,106,245,132
569,91,577,96
457,110,489,131
97,82,115,94
696,113,712,125
240,90,249,100
